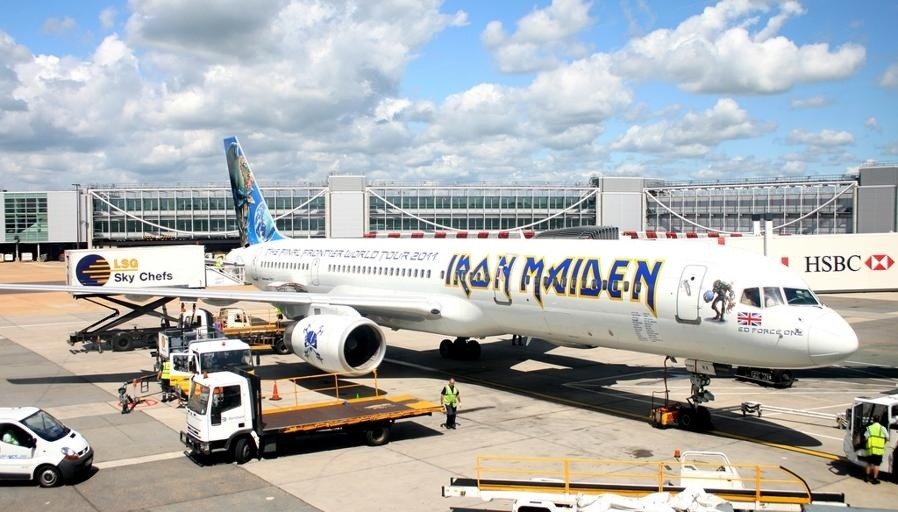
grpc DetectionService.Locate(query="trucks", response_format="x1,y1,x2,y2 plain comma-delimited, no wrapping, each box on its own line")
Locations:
833,390,897,483
435,452,745,512
70,305,252,353
158,325,256,401
178,362,447,468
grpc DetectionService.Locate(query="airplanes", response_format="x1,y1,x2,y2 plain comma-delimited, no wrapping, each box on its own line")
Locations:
0,132,862,433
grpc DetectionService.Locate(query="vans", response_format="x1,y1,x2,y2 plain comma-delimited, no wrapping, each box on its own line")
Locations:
1,405,95,489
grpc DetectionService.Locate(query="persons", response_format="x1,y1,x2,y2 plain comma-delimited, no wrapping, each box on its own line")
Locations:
275,307,283,320
863,413,890,485
441,376,462,429
156,358,174,402
710,279,736,324
173,360,188,402
512,334,524,346
